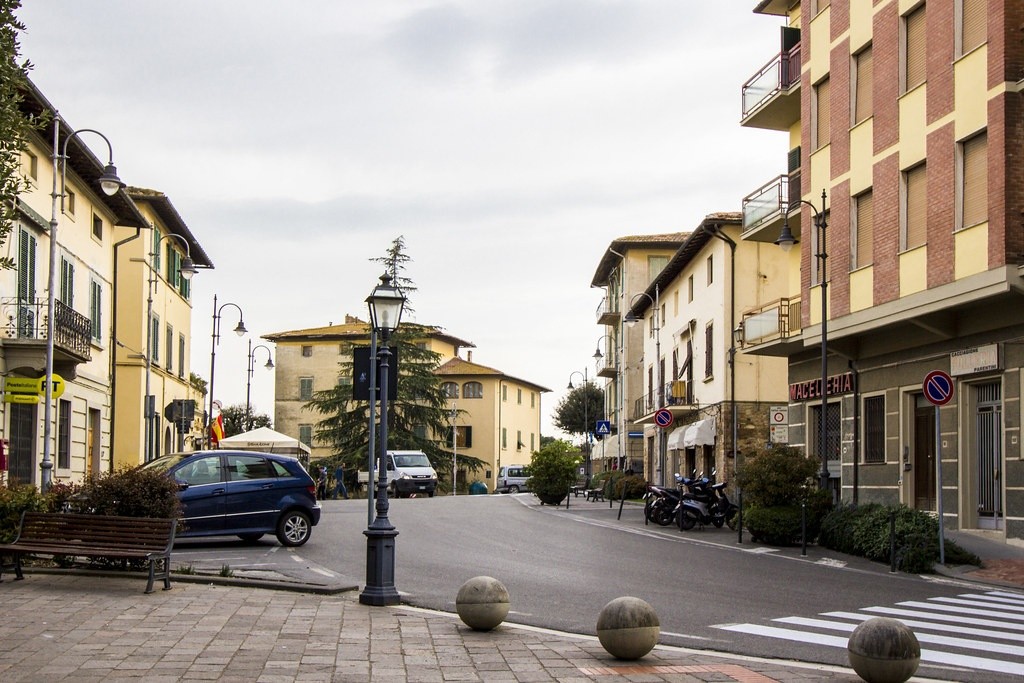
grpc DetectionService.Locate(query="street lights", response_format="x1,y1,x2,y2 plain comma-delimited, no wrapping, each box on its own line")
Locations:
773,188,830,500
626,284,663,488
567,366,589,478
207,294,249,451
247,338,275,432
128,224,199,462
591,323,620,470
19,109,127,498
355,269,408,613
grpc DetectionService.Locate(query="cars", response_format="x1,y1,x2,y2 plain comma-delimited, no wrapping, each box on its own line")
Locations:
55,448,323,547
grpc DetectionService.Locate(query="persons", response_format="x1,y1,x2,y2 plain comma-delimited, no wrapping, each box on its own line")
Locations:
332,462,351,500
316,467,327,500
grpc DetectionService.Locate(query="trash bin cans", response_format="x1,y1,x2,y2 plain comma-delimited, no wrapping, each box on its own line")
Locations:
468,481,487,494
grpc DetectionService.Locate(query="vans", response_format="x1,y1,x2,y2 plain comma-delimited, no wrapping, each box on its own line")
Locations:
497,465,538,494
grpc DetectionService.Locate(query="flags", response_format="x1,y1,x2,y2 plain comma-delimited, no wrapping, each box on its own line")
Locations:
211,413,225,443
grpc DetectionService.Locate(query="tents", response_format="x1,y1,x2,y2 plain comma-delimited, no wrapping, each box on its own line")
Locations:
218,427,311,472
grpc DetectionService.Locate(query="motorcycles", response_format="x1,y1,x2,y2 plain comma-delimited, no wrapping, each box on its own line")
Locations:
643,468,704,526
671,466,745,531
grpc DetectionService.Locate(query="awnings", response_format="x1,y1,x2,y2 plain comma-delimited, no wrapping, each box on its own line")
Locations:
590,434,625,460
667,418,714,451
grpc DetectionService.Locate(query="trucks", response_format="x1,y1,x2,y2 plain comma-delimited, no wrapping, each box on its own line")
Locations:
357,449,439,498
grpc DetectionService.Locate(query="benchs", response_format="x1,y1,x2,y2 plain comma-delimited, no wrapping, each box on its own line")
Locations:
570,478,606,503
0,511,179,594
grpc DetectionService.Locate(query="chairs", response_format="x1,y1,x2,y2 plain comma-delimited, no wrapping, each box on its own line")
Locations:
191,461,210,485
209,461,221,483
